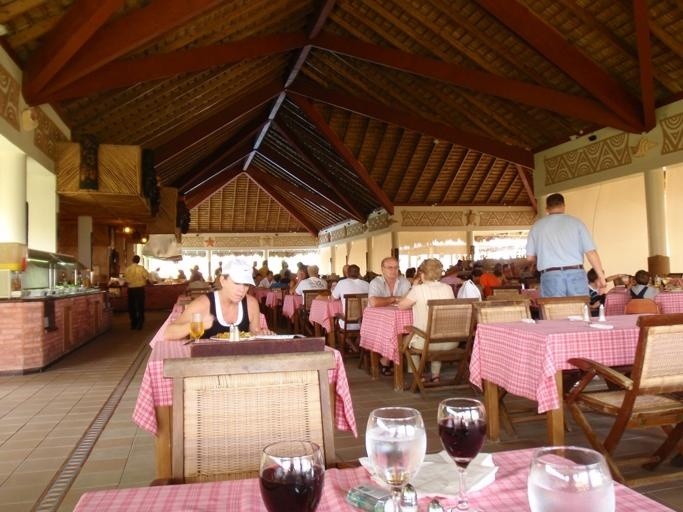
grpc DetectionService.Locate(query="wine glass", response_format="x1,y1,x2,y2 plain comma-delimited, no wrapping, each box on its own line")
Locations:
436,395,484,509
363,406,426,512
188,311,203,343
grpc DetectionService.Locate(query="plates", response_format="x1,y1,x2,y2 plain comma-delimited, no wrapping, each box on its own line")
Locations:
209,335,257,341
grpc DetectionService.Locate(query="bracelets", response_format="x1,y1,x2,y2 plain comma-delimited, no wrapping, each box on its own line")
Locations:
391,296,396,304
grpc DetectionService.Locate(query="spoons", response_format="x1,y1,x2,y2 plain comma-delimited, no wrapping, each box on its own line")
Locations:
397,481,447,512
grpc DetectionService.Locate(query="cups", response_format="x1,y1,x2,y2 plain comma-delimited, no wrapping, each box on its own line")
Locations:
255,440,326,512
527,444,616,512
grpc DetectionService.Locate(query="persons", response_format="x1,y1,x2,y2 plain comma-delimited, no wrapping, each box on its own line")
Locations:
252,259,513,384
165,257,276,343
628,270,660,301
587,269,605,306
146,260,224,286
124,254,150,328
526,193,606,298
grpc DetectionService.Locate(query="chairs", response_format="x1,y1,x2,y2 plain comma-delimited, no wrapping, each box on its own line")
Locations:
561,314,682,488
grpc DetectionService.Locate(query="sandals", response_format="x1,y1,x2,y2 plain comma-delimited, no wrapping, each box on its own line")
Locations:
379,359,440,383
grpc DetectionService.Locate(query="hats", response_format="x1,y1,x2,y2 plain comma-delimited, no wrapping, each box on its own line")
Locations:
222,255,256,286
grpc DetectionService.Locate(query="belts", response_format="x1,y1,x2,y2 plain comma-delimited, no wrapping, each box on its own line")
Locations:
541,265,584,273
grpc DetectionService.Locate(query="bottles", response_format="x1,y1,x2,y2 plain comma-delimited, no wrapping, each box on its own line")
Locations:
228,323,239,340
347,484,398,511
582,303,607,323
